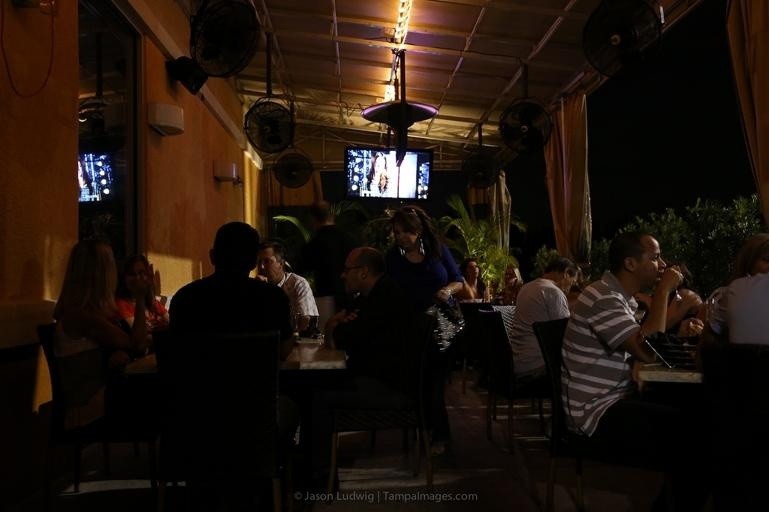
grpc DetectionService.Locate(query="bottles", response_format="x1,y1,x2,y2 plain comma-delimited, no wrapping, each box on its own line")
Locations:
484,281,494,303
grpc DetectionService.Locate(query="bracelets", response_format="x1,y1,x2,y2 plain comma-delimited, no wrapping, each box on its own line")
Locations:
441,286,454,297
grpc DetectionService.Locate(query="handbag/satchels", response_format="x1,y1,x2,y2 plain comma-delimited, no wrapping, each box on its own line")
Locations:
425,292,466,353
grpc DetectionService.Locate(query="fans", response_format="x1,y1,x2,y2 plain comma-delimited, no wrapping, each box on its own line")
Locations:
164,2,261,96
457,123,500,190
243,35,297,155
275,95,314,189
580,1,663,80
498,62,553,157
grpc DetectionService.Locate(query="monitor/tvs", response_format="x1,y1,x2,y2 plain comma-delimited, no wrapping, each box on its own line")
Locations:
344,145,433,202
77,151,113,209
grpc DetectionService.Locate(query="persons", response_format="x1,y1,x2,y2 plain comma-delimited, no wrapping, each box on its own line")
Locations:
165,217,297,511
299,245,444,511
53,240,151,438
386,204,467,363
558,232,686,511
253,241,320,336
111,253,169,352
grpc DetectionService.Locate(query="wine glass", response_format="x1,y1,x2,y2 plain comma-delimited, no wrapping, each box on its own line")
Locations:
669,265,684,304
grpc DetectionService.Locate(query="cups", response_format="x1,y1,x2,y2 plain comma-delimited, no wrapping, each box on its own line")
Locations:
460,299,483,303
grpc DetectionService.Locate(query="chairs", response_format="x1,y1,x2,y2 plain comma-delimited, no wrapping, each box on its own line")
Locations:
37,320,282,511
679,345,768,511
327,311,440,493
464,297,591,512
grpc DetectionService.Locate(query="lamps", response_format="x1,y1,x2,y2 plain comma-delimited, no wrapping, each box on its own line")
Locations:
211,158,240,183
151,100,188,139
